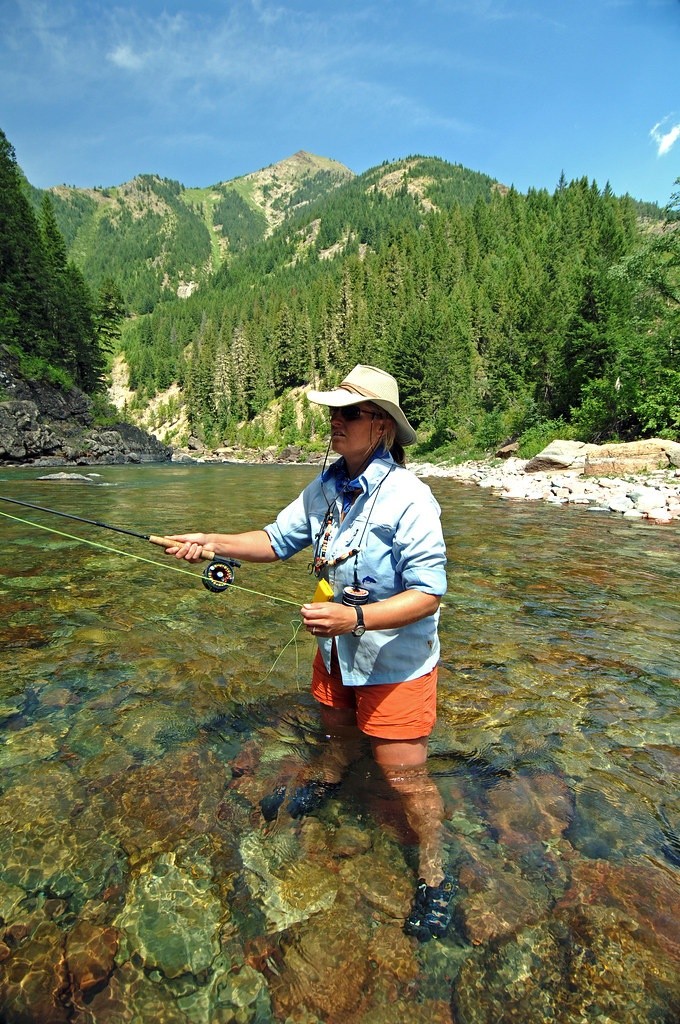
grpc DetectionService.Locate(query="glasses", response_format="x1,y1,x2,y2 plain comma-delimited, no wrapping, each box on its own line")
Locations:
328,405,385,421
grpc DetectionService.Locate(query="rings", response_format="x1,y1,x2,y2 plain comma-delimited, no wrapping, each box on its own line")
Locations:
312,627,315,634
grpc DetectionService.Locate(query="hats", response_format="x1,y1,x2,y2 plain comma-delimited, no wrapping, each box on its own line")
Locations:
306,364,417,447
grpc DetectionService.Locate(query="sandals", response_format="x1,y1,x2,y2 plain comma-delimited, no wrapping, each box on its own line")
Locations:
405,875,459,942
258,779,340,822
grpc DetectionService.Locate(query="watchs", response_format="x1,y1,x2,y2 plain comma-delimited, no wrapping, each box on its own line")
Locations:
351,606,365,637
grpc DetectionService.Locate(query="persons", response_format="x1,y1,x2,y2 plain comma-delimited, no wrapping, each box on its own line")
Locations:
163,365,448,766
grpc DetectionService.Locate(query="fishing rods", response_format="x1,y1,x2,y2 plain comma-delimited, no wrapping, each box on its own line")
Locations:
1,495,244,595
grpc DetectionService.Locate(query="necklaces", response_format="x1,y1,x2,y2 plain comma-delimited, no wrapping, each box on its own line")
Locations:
307,483,380,577
